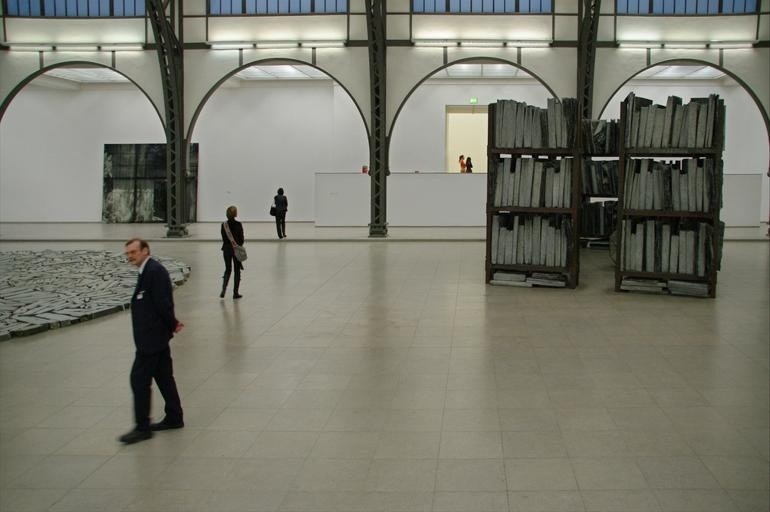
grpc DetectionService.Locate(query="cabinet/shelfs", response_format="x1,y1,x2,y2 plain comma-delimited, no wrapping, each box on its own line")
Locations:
614,100,724,299
579,152,621,249
485,99,585,290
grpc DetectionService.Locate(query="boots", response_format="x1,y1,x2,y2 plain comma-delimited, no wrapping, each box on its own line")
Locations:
220,277,229,297
233,279,243,299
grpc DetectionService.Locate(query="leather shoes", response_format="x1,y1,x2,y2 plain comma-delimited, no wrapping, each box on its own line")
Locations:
120,422,152,443
153,415,184,430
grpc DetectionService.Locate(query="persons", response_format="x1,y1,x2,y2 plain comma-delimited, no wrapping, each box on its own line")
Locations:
220,206,244,299
458,155,466,172
274,188,288,238
117,237,184,443
466,157,473,172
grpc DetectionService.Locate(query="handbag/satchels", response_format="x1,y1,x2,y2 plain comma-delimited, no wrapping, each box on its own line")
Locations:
270,206,277,216
233,245,247,262
